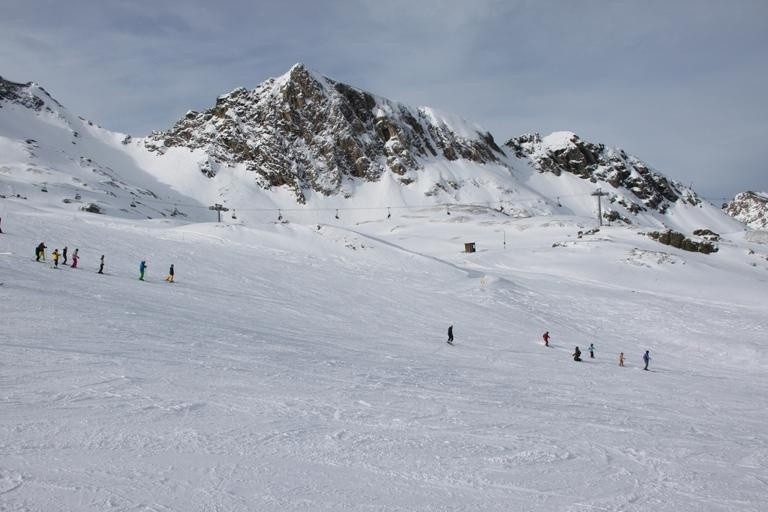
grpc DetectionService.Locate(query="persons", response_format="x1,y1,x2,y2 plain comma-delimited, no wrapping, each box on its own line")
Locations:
41,241,46,260
587,342,597,359
542,330,550,345
139,260,147,281
619,350,627,367
572,346,582,362
97,254,105,274
34,243,47,262
50,248,61,269
61,245,69,266
642,348,651,371
69,249,79,269
165,264,175,283
447,324,454,343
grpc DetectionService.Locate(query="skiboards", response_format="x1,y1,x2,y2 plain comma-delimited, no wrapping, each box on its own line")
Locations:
31,258,47,263
547,345,554,349
50,266,65,270
642,369,656,373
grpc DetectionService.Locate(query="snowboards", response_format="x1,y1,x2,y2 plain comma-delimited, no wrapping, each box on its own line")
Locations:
447,341,454,346
